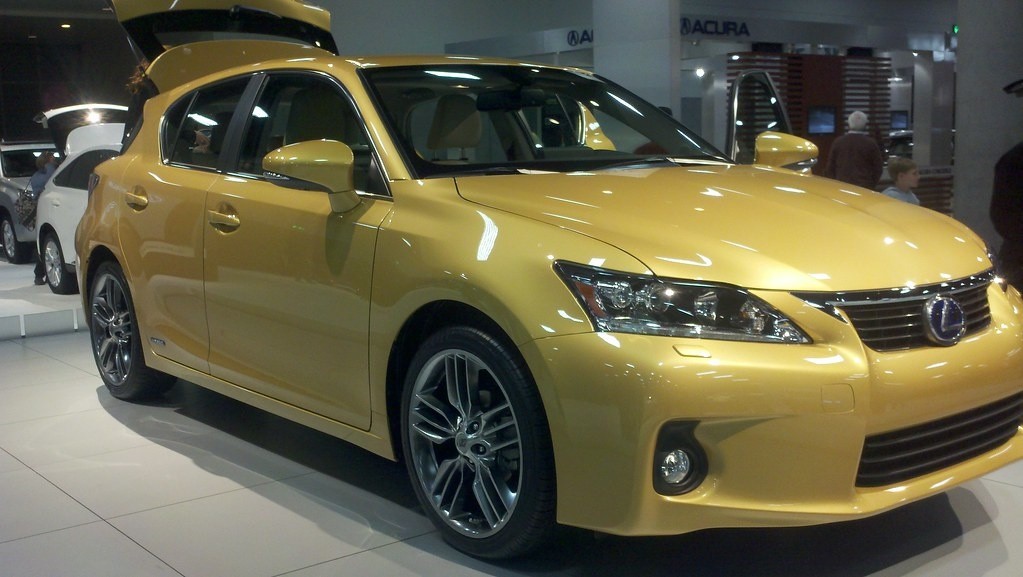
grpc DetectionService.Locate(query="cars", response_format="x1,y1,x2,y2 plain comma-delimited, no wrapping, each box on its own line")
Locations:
0,135,69,265
66,0,1022,570
31,103,138,296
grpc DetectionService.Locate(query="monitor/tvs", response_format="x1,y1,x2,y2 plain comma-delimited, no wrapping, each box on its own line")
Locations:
890,111,908,130
807,105,837,134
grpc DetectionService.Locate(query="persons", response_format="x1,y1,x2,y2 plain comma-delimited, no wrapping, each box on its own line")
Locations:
882,158,920,206
634,107,673,155
990,141,1023,293
31,151,59,285
122,46,211,163
825,111,883,189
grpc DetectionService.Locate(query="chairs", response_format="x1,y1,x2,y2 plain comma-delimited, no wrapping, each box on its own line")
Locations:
425,93,481,165
208,111,273,155
283,90,347,142
